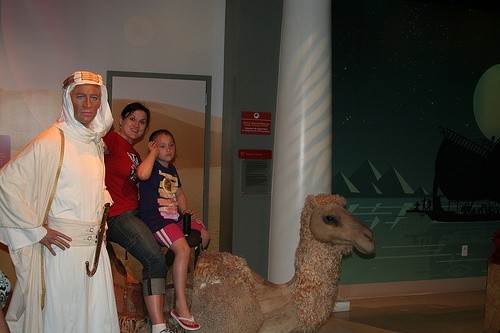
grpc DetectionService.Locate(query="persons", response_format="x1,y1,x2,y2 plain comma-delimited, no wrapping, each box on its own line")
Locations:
0,71,121,333
135,129,210,330
101,102,206,333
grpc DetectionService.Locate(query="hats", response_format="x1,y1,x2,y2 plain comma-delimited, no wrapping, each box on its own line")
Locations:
51,71,114,144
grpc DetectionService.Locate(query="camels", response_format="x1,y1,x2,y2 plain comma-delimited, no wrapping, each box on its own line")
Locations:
0,193,377,333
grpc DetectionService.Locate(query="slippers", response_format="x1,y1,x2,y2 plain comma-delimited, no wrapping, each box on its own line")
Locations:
170,309,202,331
